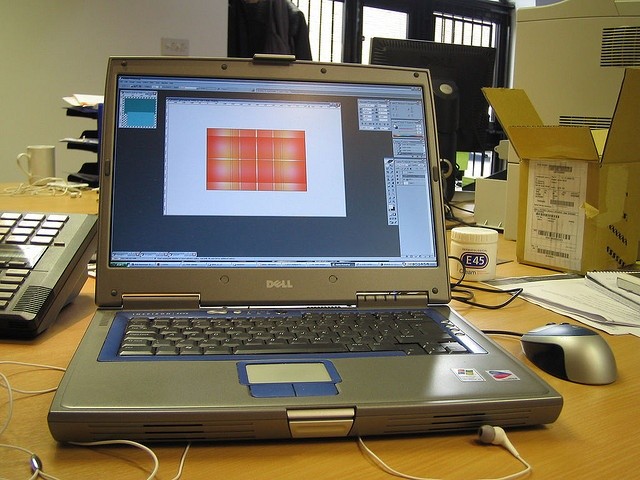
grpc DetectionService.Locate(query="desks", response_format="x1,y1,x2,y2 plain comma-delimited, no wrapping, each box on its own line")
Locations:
0,181,636,480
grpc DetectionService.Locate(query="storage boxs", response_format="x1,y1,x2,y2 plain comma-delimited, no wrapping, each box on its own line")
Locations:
480,68,640,277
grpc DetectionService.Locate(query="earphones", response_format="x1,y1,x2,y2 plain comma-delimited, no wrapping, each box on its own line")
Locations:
478,424,522,459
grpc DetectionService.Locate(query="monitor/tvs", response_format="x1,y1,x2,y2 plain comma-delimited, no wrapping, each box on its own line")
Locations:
369,37,497,203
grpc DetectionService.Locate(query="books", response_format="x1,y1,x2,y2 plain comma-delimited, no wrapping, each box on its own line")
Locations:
580,264,640,313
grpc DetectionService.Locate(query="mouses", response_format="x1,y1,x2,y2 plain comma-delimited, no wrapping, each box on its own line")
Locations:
520,322,617,386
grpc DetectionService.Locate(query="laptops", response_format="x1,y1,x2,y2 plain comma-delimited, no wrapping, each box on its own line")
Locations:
47,54,564,444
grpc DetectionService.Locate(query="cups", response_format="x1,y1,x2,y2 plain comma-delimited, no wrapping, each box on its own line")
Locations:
15,144,56,188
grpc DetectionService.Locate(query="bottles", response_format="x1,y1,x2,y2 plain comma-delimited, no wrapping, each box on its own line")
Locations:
449,227,499,282
